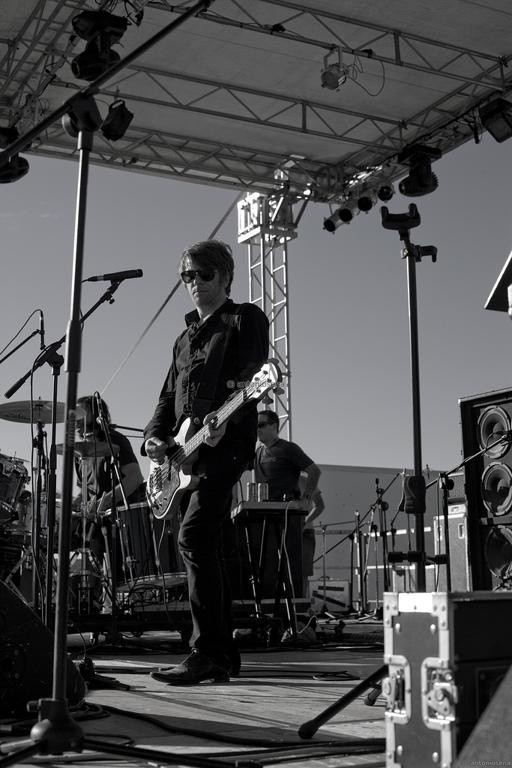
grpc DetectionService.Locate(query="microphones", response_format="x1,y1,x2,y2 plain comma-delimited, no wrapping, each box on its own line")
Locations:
88,269,143,283
39,311,45,350
96,395,104,416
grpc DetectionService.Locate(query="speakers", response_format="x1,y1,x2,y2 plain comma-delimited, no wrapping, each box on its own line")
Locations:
458,387,512,592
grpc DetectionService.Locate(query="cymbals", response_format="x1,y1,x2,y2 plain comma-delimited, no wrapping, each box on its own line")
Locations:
0,401,86,423
56,443,119,459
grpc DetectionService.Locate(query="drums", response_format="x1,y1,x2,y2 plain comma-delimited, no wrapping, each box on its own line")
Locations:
104,502,188,588
53,548,103,615
0,454,30,519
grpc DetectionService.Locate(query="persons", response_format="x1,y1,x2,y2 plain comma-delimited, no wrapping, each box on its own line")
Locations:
74,395,147,587
235,409,321,647
294,471,326,598
138,236,271,688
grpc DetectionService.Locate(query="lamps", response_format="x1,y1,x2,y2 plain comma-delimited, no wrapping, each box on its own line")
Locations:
2,8,139,189
319,163,397,238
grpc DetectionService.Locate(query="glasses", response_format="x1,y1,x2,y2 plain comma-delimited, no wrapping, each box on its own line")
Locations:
257,421,271,430
179,268,220,284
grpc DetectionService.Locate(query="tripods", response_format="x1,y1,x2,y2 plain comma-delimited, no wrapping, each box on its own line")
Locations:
312,519,389,624
4,419,80,607
297,229,436,739
0,127,263,768
40,458,123,615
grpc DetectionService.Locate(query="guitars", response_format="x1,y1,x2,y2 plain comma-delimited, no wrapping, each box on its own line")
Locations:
145,359,283,520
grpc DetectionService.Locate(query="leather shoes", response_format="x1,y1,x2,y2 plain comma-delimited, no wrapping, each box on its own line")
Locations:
150,644,242,686
